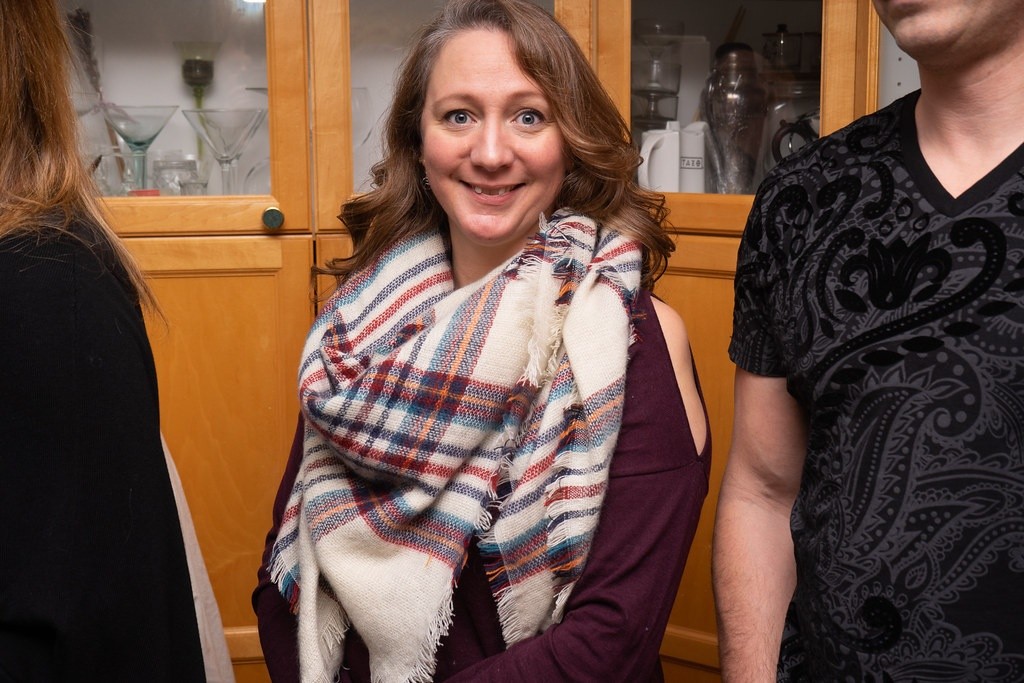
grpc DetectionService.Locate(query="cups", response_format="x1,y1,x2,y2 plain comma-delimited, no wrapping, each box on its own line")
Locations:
637,129,680,192
100,152,210,194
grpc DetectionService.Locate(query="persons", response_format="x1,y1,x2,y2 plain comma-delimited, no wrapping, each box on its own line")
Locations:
709,0,1024,683
250,0,711,683
0,0,235,683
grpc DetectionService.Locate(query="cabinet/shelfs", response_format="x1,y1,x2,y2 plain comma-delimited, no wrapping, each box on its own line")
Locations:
47,0,881,683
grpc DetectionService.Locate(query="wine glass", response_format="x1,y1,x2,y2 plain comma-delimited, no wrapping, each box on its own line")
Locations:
181,108,269,195
98,103,178,189
